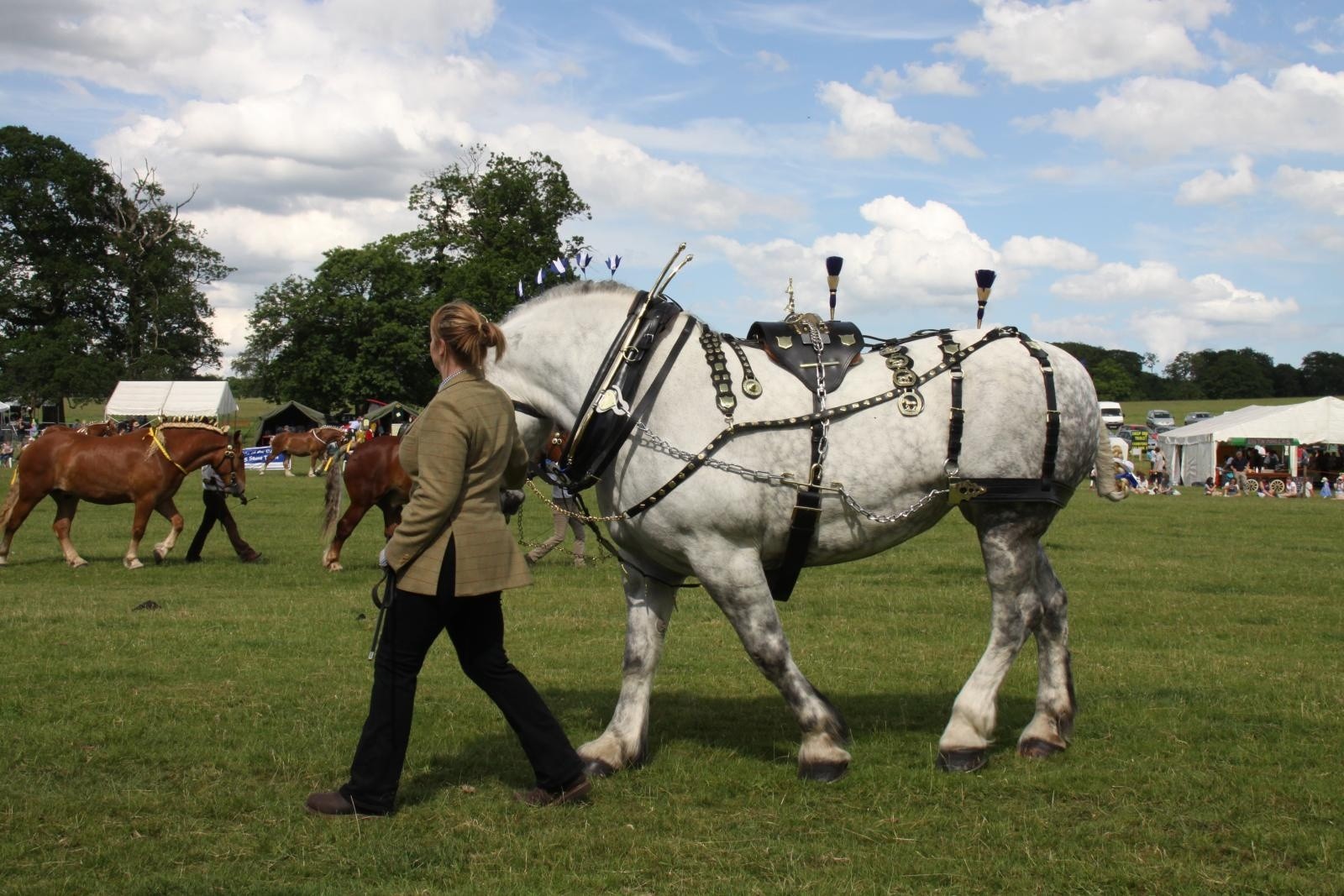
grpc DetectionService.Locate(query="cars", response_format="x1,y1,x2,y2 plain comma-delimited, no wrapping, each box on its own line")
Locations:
1146,409,1176,432
1183,412,1211,426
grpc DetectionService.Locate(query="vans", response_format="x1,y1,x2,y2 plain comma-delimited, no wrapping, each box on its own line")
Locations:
1097,401,1126,429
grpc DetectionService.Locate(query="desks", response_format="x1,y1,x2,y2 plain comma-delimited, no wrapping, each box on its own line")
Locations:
1245,472,1291,483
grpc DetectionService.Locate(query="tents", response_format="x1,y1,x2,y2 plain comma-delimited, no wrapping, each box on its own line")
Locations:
1155,395,1344,487
363,400,421,436
243,400,326,448
103,381,239,434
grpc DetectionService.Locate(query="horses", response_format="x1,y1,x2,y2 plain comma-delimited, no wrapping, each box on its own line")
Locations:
0,422,246,569
259,425,347,477
38,420,119,440
481,279,1131,780
322,435,413,573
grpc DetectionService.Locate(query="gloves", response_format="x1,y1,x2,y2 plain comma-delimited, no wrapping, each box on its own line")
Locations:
379,548,391,571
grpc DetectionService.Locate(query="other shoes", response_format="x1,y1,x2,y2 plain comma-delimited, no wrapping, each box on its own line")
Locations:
513,776,593,808
186,556,200,561
304,791,363,816
244,550,263,563
524,553,535,567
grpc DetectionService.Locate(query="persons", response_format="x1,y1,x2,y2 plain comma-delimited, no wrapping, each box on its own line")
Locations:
184,464,262,566
1090,445,1344,503
343,416,362,472
524,462,586,569
306,300,594,822
0,416,82,468
